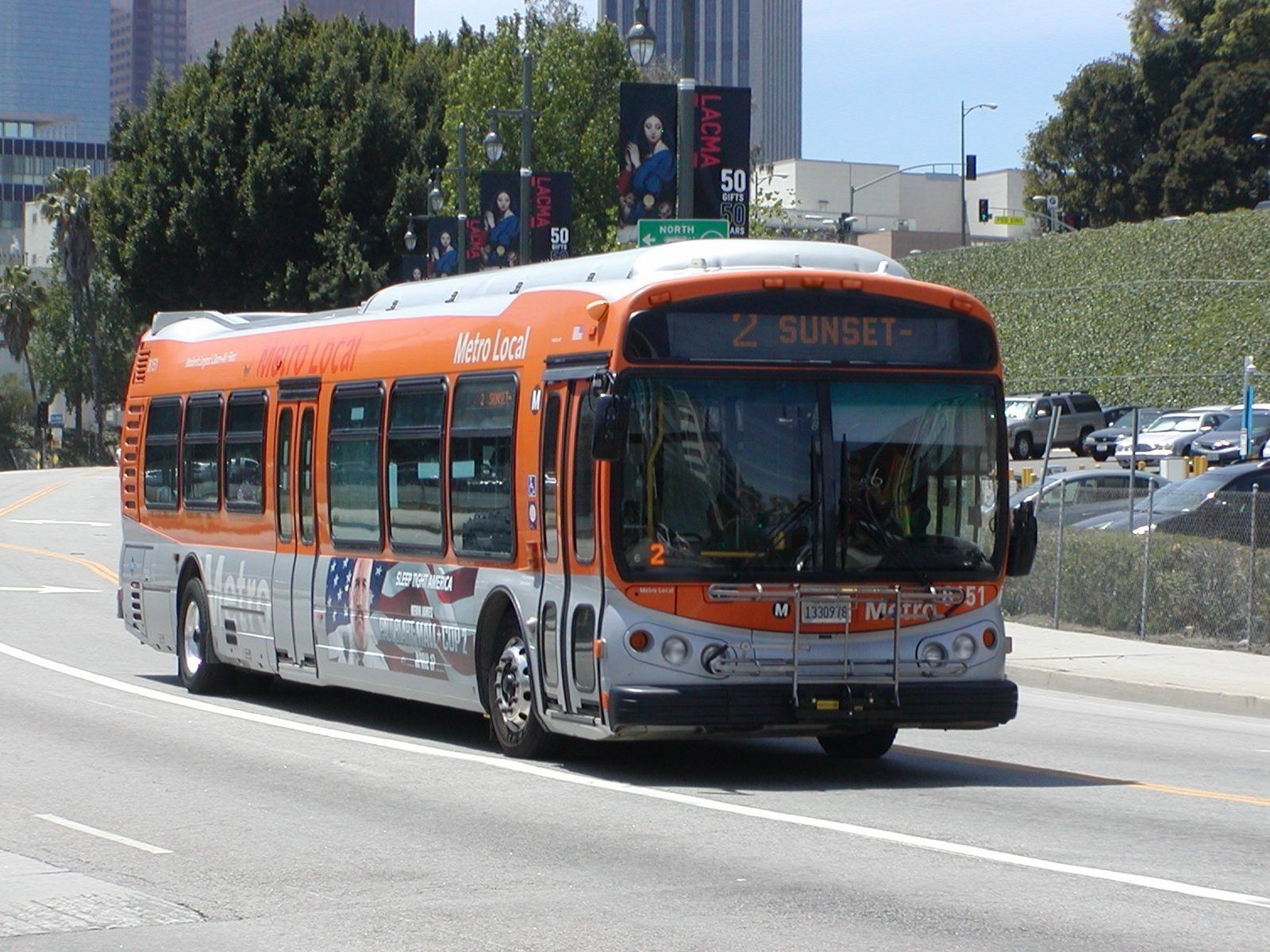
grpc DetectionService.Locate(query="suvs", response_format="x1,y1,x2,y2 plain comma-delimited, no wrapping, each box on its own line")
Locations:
1004,388,1106,461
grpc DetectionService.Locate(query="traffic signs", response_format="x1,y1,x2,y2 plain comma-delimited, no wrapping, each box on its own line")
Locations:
637,218,730,247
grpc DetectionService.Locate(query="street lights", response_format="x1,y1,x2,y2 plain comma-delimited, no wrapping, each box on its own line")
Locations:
483,47,535,265
425,120,467,275
754,171,789,219
1032,196,1055,232
403,178,434,276
626,0,697,220
961,99,998,247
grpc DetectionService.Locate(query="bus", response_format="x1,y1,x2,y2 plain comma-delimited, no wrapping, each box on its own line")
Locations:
113,236,1041,764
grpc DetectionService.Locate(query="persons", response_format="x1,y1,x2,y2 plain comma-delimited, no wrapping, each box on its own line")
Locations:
407,189,519,284
327,558,391,671
623,111,677,226
815,457,902,557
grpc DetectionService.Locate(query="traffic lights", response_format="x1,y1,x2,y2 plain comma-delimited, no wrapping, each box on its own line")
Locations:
978,198,988,223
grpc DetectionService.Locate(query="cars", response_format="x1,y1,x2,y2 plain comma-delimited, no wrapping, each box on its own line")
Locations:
1081,403,1270,469
